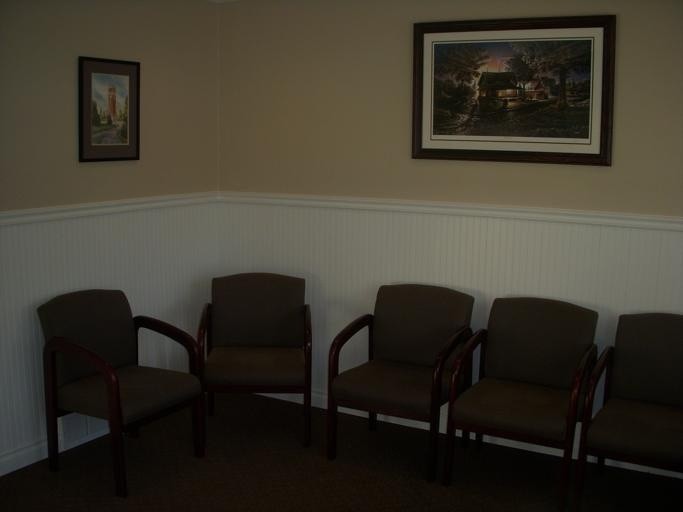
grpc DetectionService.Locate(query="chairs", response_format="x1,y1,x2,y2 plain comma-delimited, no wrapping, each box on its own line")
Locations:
439,295,598,493
37,288,210,510
588,313,681,510
328,282,473,489
197,273,314,456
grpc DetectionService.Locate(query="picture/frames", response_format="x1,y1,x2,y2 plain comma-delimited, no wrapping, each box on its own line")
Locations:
76,54,141,164
410,14,617,168
